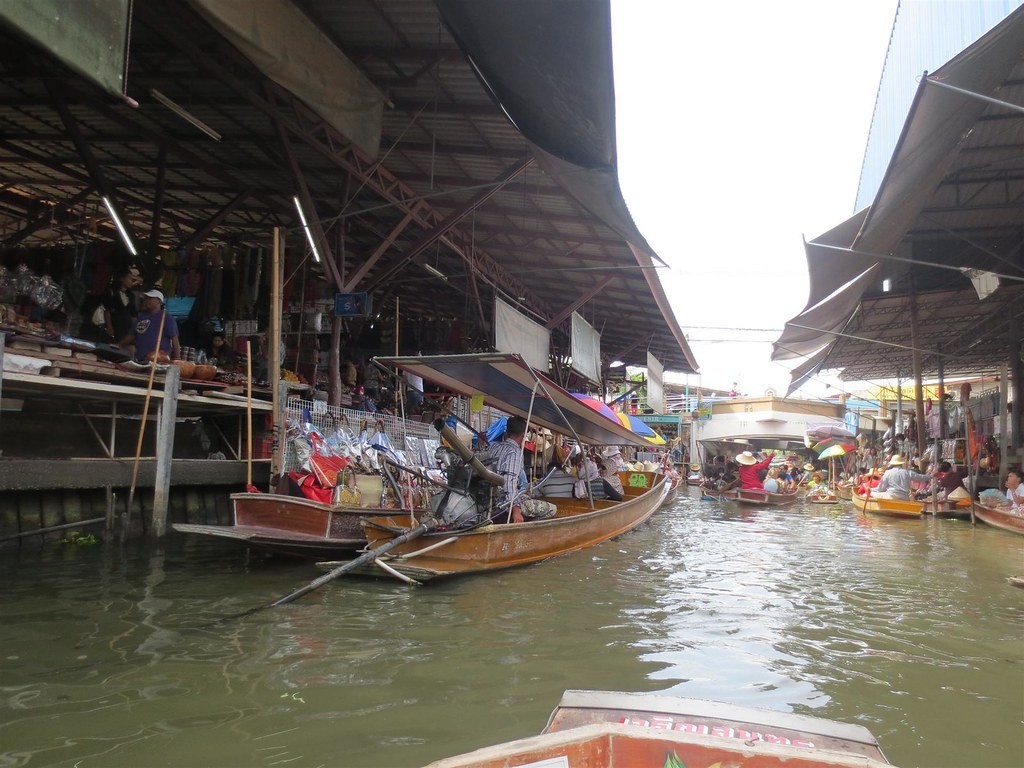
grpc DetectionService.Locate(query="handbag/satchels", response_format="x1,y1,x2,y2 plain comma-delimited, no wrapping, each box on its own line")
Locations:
280,407,430,510
91,286,114,328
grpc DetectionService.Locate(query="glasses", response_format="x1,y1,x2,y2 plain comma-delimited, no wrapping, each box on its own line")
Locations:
598,464,607,470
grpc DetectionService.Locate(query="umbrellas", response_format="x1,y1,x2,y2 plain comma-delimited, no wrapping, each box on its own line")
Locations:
570,391,624,428
641,423,667,445
616,412,657,438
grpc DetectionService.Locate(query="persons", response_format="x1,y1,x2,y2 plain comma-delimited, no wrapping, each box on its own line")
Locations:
361,358,384,406
657,430,668,453
201,331,235,371
117,289,181,365
937,457,968,500
96,266,139,345
729,388,737,400
484,415,531,525
671,434,680,462
987,469,1024,517
667,434,673,454
529,443,625,503
407,388,424,414
629,391,640,415
700,447,938,500
340,355,357,391
256,325,286,386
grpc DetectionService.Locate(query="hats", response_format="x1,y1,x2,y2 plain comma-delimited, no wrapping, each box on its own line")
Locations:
145,289,164,304
627,461,644,472
888,454,905,465
810,471,823,479
948,486,971,506
803,463,814,471
691,464,701,471
736,451,757,465
643,461,659,473
601,445,622,457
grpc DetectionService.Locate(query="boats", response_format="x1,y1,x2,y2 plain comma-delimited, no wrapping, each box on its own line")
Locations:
314,350,675,582
664,430,1024,538
421,688,895,767
170,455,449,564
